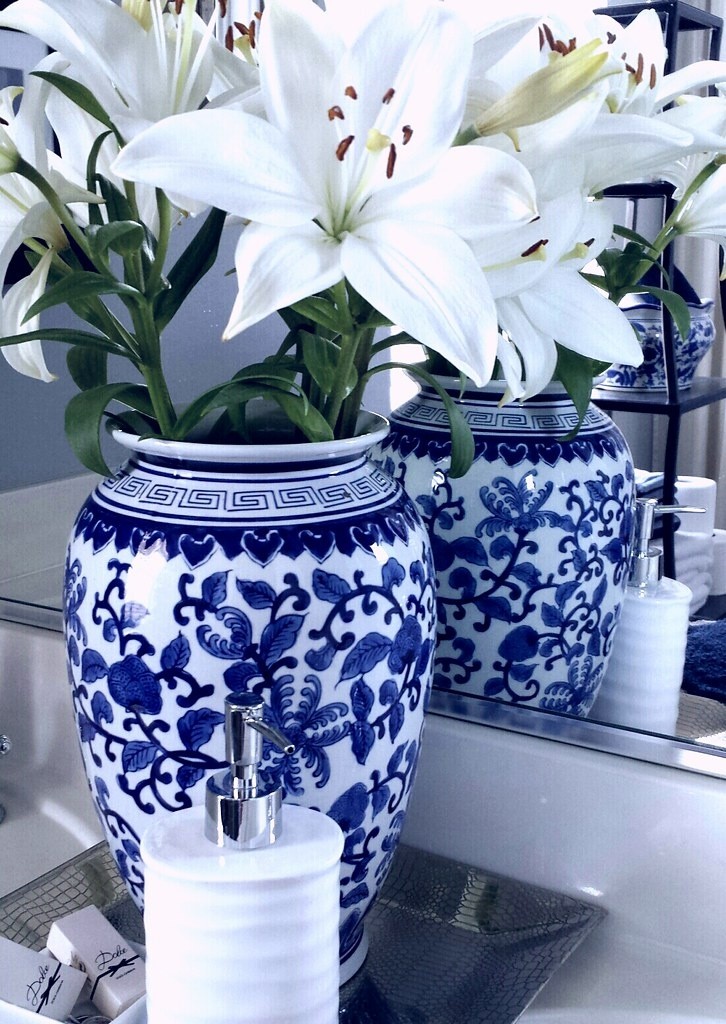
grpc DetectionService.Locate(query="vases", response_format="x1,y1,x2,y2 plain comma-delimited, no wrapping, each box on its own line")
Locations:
590,289,719,393
58,413,433,989
366,357,642,718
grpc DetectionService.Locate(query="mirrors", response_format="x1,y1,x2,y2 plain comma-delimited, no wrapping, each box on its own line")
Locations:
0,0,726,779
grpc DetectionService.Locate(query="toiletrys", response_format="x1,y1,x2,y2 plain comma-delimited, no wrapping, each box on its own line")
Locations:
136,693,345,1022
581,495,706,736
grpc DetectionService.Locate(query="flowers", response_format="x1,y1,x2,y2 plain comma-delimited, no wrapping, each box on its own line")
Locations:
0,0,690,480
414,10,699,441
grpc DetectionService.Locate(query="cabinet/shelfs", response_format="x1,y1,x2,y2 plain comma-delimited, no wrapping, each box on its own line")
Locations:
589,0,725,577
